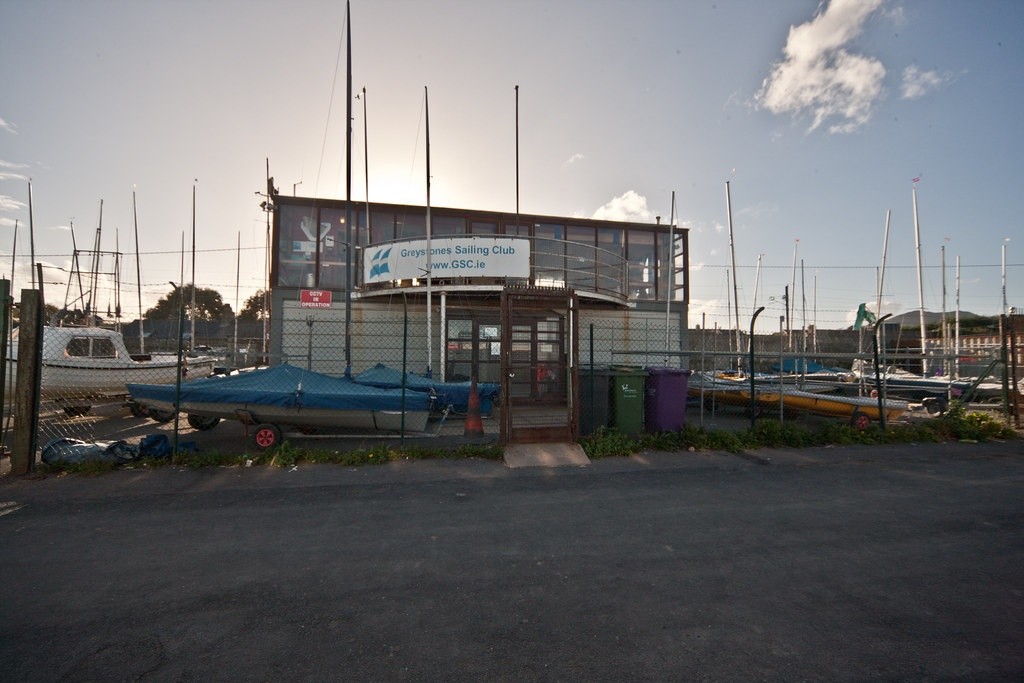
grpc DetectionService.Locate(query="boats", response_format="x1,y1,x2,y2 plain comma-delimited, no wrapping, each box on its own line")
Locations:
343,360,502,414
125,361,433,435
3,325,212,397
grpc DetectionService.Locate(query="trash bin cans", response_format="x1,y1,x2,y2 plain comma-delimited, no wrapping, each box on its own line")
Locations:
608,365,649,435
570,364,611,437
645,366,692,433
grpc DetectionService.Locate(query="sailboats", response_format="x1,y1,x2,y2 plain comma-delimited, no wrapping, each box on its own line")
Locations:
683,177,1015,418
